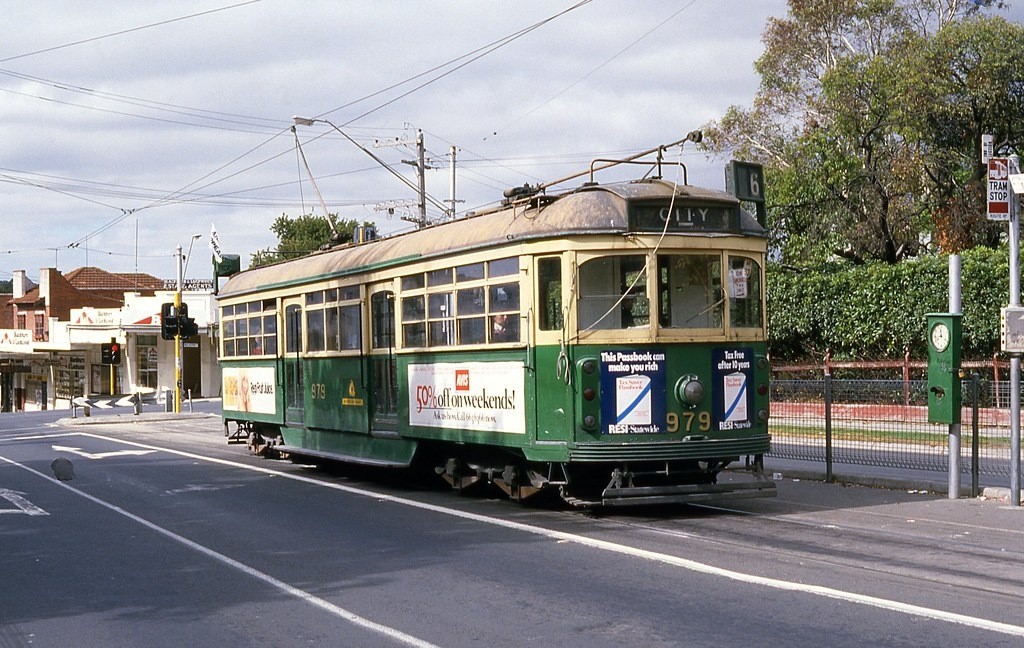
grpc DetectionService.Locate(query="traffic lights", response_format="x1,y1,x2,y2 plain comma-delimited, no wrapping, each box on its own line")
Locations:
183,303,198,338
101,343,111,364
162,303,176,340
112,343,120,364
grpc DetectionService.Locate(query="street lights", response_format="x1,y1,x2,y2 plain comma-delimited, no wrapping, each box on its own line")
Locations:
177,235,202,401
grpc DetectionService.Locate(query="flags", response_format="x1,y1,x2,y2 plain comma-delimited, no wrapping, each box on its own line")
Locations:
209,224,222,263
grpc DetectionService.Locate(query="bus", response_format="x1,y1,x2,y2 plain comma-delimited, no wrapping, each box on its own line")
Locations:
216,156,778,511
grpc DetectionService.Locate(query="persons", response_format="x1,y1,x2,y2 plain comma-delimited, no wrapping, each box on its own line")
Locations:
491,305,517,342
252,331,269,355
620,288,636,328
